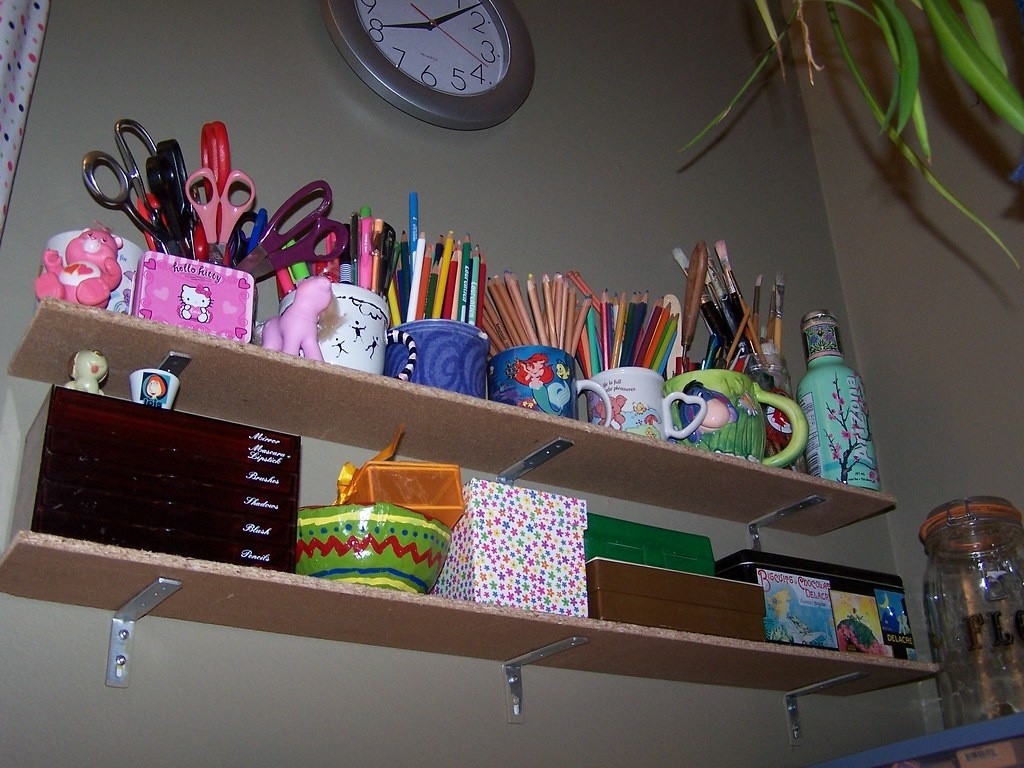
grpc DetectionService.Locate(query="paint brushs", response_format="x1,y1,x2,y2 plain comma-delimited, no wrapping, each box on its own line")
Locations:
673,239,786,374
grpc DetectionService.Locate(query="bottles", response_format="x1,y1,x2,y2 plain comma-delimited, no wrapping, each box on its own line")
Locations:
796,309,880,490
920,495,1024,732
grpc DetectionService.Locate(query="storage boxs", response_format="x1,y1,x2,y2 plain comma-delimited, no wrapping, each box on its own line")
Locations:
715,549,918,661
584,512,715,577
346,461,465,530
8,384,302,573
429,477,588,618
585,556,766,642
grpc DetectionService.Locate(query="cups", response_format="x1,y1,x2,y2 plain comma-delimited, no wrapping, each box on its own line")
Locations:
279,283,389,376
43,231,141,316
490,345,611,428
385,319,489,400
587,366,708,443
666,369,809,467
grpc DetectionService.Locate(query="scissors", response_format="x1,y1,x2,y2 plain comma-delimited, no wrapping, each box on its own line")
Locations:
237,179,350,281
136,139,267,268
199,121,233,266
80,117,186,257
185,168,256,267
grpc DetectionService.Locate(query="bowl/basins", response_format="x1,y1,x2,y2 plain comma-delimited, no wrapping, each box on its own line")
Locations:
296,503,451,595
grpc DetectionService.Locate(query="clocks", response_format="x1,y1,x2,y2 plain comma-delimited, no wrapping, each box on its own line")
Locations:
319,0,536,130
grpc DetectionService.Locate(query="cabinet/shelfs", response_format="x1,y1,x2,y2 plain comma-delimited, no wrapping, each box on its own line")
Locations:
0,297,941,746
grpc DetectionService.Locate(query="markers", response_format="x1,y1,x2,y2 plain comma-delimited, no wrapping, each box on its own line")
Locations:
390,192,487,332
577,288,680,379
480,268,593,362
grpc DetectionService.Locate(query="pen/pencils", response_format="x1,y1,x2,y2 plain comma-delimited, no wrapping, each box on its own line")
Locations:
273,206,398,299
